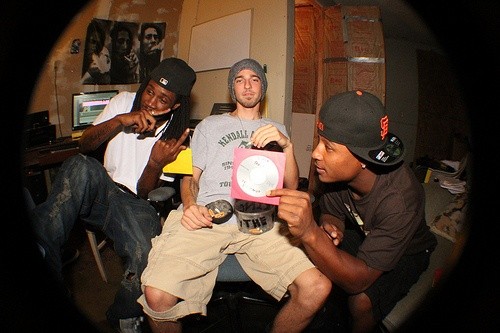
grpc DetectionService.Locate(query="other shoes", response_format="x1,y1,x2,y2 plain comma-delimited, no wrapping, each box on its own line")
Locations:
105,310,152,333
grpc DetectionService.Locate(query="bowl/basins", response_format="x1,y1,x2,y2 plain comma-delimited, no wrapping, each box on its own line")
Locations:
233,200,275,235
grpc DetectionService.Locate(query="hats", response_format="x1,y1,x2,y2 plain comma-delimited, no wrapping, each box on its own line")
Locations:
316,90,406,166
149,57,196,96
228,57,268,104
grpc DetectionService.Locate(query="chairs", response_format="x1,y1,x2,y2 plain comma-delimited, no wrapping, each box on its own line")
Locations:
77,215,126,282
146,168,292,317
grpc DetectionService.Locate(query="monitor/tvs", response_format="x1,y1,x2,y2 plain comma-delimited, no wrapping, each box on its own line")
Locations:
72,90,119,131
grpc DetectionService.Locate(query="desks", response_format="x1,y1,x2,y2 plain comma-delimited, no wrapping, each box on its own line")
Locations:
19,132,104,201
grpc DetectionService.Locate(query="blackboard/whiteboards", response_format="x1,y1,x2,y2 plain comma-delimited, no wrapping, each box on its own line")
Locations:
188,8,254,73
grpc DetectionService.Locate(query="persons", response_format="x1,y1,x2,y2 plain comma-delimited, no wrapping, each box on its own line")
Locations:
84,18,164,85
137,59,332,333
18,56,198,333
265,89,437,333
